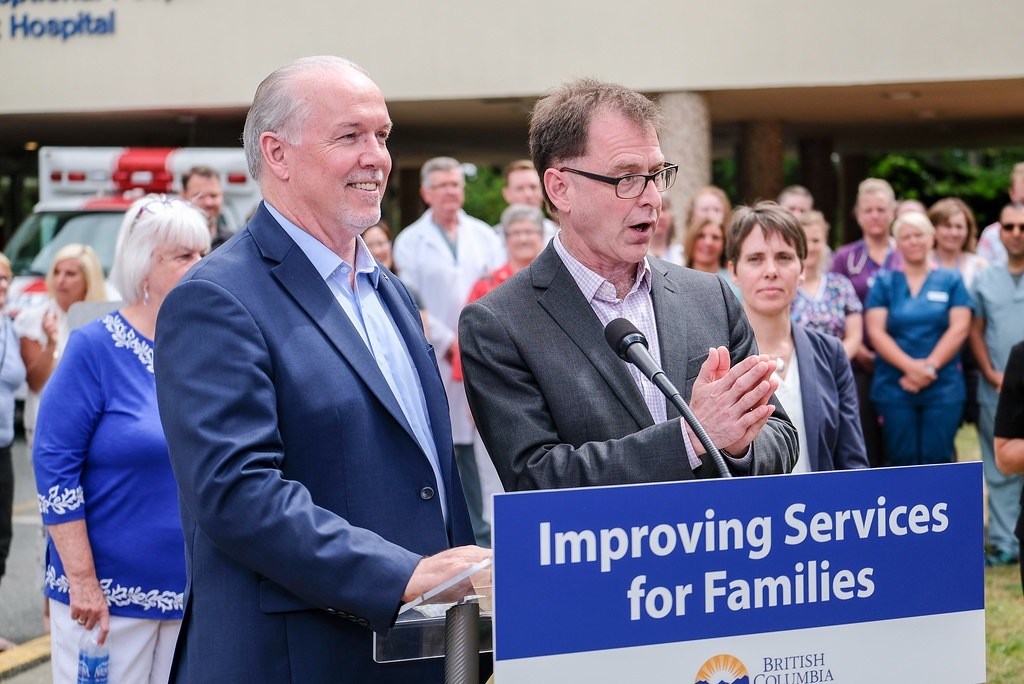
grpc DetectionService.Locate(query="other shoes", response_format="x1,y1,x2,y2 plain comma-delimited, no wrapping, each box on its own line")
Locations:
984,547,1019,567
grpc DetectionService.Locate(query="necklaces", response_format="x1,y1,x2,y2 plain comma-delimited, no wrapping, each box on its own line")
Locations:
774,356,784,372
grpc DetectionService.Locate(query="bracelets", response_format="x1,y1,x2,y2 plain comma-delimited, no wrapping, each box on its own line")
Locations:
928,367,938,374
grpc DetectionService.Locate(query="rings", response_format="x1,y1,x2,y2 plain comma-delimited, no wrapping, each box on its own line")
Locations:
78,618,85,623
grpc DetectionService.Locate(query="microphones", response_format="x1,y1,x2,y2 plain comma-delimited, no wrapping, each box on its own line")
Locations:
604,318,731,478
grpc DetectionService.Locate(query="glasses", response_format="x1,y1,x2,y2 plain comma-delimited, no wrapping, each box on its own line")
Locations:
130,192,185,238
559,162,678,199
1000,221,1024,231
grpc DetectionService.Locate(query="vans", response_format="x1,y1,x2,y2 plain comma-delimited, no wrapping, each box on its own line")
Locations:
1,145,261,283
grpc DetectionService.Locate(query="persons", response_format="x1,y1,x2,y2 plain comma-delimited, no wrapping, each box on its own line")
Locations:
181,167,234,252
0,243,108,631
33,195,210,684
649,187,743,303
726,201,869,474
458,80,800,491
992,341,1024,593
361,158,559,546
781,164,1024,564
154,55,492,684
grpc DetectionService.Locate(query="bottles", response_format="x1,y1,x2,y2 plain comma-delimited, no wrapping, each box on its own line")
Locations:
76,620,109,684
